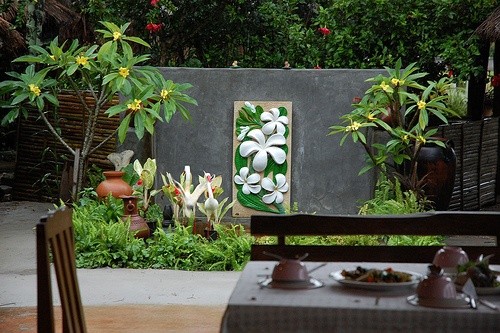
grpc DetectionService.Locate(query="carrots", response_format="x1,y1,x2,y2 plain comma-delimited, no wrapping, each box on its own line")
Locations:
384,268,394,273
368,276,375,282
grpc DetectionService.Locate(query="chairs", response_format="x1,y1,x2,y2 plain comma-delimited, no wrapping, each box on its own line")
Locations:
36,206,87,333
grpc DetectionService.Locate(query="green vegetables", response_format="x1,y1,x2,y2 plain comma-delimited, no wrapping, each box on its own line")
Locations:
456,257,489,273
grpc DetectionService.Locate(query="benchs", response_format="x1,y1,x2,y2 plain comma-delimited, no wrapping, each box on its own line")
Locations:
250,211,500,264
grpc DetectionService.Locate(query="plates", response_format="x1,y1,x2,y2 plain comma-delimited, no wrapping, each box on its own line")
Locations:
257,276,323,289
406,294,470,308
329,269,428,290
426,270,500,295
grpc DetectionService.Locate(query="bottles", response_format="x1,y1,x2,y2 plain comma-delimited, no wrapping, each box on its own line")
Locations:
95,170,132,204
118,195,150,241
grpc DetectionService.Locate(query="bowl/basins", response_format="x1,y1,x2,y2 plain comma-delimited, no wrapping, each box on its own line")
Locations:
415,274,457,299
433,246,469,269
271,260,308,282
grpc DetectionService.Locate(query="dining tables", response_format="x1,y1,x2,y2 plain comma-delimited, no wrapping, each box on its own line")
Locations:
218,261,500,333
